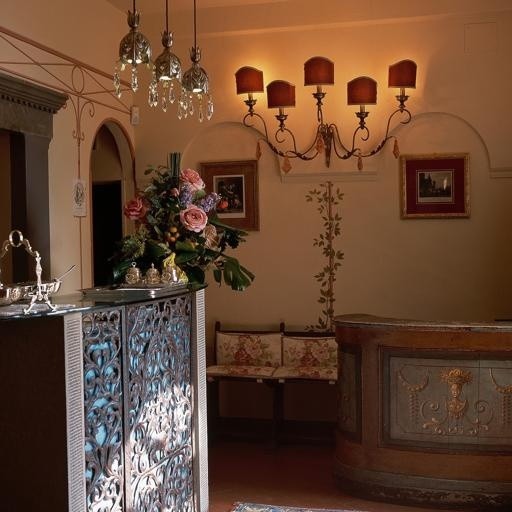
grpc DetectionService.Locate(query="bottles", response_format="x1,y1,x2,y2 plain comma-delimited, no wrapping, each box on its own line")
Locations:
125,260,177,285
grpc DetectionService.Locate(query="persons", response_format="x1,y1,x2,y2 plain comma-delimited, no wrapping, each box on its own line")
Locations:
445,383,467,418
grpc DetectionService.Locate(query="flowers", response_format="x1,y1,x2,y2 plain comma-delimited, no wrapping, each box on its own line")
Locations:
123,163,256,291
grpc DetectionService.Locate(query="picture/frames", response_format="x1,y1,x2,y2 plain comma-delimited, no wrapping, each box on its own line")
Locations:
399,152,470,219
197,159,261,232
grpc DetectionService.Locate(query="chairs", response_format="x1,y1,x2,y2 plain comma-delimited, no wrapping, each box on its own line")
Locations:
206,320,337,448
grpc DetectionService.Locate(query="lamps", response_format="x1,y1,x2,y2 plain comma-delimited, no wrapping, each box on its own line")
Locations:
234,56,417,168
112,0,215,123
